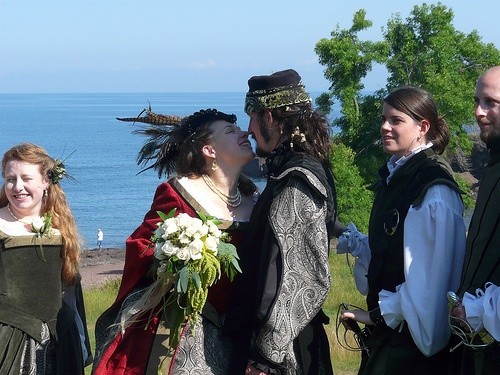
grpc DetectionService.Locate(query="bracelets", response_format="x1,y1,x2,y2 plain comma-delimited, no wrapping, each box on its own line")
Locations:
370,309,383,326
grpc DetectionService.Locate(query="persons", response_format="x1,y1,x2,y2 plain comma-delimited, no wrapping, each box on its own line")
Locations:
92,109,260,375
450,67,500,375
0,144,94,375
227,70,335,375
96,229,103,252
329,88,466,375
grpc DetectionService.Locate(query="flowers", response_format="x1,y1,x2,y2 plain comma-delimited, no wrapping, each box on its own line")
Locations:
31,215,52,262
143,208,243,375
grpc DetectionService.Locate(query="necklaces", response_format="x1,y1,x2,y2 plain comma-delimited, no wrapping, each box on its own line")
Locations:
201,174,242,208
8,204,31,226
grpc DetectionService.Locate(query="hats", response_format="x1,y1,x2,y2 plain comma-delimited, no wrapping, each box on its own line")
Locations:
244,68,312,115
